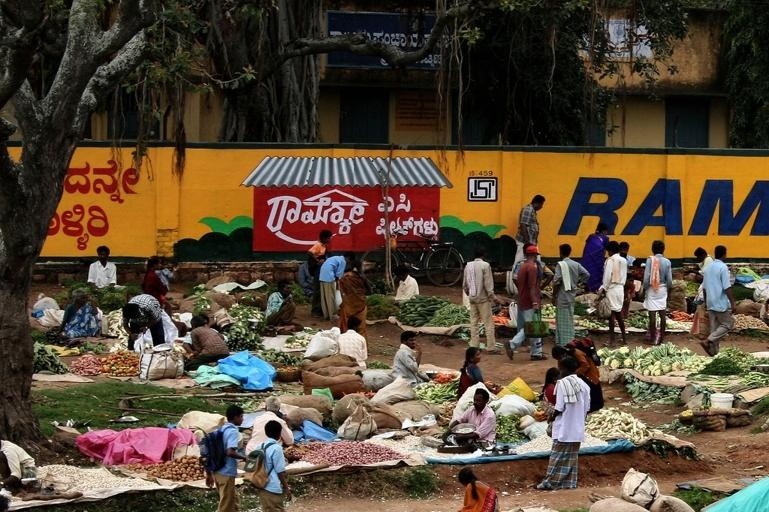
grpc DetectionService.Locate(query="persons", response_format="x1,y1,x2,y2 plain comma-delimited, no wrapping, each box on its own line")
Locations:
252,420,292,512
462,245,506,355
307,230,332,318
504,246,549,361
190,315,229,363
392,330,429,385
517,194,545,246
643,240,673,345
200,315,225,342
59,289,98,336
442,387,497,448
512,242,555,334
263,279,296,325
0,438,36,490
122,293,166,350
245,396,294,457
582,223,608,260
699,245,736,356
87,246,117,293
204,404,245,512
142,257,172,317
541,367,560,405
456,346,503,399
552,344,605,414
338,260,371,338
337,317,368,370
393,265,419,303
597,241,628,346
550,243,590,346
694,247,714,277
318,250,355,321
534,357,591,489
456,466,499,512
619,241,635,276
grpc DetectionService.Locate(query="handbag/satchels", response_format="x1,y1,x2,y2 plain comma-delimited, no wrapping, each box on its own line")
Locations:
524,319,550,337
690,304,716,340
596,295,613,319
138,347,186,382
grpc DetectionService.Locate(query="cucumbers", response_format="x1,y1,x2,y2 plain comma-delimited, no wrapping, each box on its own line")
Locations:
223,326,257,350
395,294,445,326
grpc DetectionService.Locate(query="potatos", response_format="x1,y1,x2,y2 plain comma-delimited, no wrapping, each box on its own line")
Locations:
144,456,205,481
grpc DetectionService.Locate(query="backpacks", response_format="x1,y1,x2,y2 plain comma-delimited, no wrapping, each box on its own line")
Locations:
564,336,599,354
194,431,228,473
243,449,270,489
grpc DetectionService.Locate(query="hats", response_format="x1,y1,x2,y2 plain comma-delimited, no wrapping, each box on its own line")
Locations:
525,246,541,256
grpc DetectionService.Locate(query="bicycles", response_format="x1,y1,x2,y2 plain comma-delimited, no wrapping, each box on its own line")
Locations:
360,232,465,287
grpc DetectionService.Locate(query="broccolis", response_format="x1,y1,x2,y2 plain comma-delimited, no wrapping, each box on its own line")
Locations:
598,342,693,376
